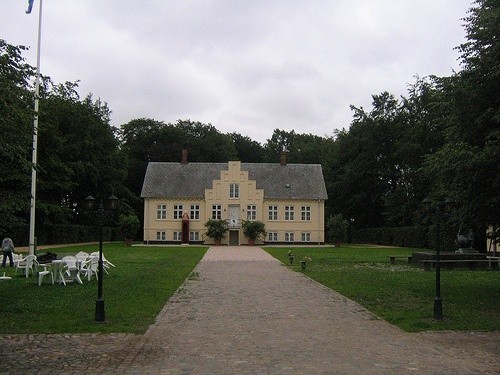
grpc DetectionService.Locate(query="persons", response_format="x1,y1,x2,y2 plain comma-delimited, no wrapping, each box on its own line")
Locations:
3,234,18,268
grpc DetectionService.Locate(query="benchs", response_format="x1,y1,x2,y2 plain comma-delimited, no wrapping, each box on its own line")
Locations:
387,254,500,271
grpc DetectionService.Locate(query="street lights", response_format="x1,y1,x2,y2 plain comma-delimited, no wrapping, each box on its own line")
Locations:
86,193,117,324
424,192,455,320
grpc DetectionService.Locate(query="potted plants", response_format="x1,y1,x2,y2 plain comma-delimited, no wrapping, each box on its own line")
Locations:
200,217,231,246
240,218,267,246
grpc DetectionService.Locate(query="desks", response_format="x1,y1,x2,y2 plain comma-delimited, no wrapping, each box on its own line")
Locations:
412,252,486,266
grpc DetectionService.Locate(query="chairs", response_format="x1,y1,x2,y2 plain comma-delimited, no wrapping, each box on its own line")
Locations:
0,252,117,287
